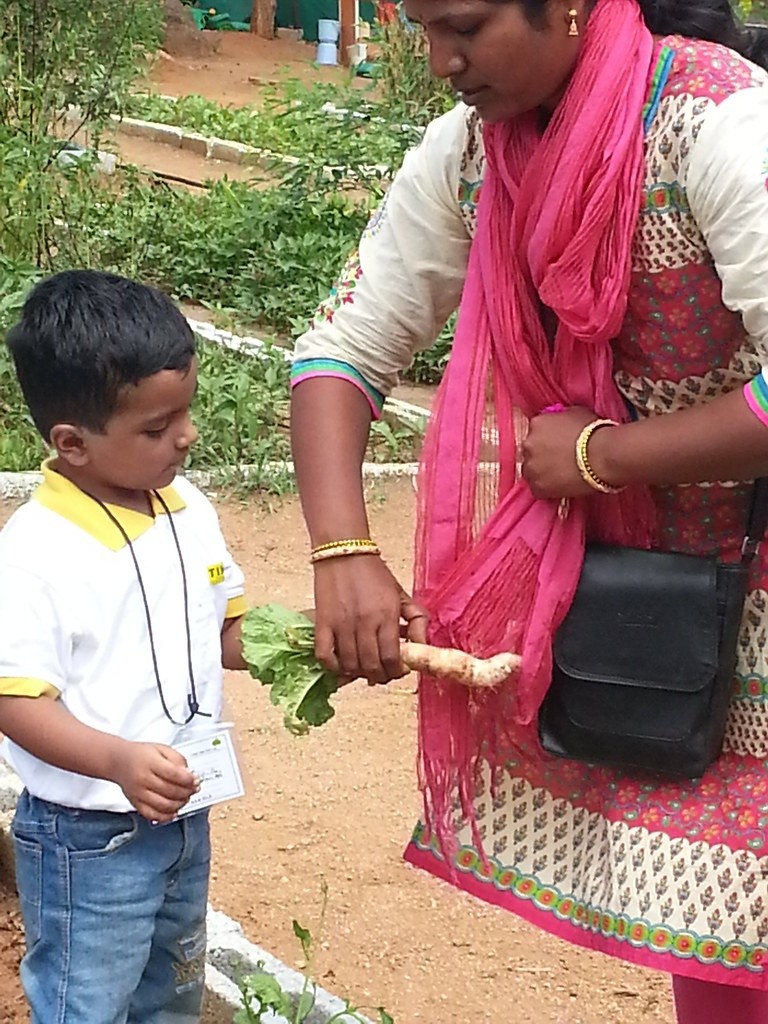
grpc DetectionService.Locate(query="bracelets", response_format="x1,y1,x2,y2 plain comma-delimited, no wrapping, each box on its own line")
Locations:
309,540,380,562
576,419,627,494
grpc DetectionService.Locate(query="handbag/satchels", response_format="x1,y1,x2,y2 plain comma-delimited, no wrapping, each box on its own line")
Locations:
538,539,748,779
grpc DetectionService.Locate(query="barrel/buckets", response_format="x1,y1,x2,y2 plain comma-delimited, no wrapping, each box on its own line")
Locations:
316,43,337,64
318,18,340,44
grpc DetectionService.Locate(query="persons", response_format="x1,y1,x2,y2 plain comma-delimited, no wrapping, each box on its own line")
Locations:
289,0,768,1023
1,269,317,1024
